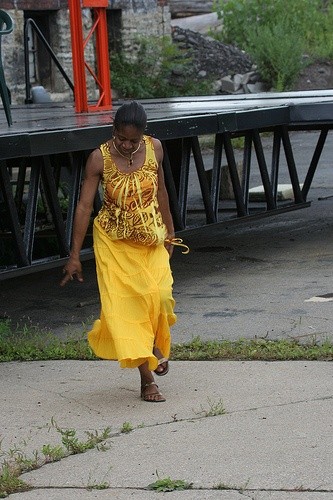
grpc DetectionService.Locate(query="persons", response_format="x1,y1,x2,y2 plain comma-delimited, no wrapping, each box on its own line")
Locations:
59,102,176,402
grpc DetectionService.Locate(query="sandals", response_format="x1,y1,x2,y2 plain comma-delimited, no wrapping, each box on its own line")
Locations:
154,348,169,376
141,382,166,402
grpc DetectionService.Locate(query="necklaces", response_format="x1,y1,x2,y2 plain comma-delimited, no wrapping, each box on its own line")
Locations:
113,136,141,168
